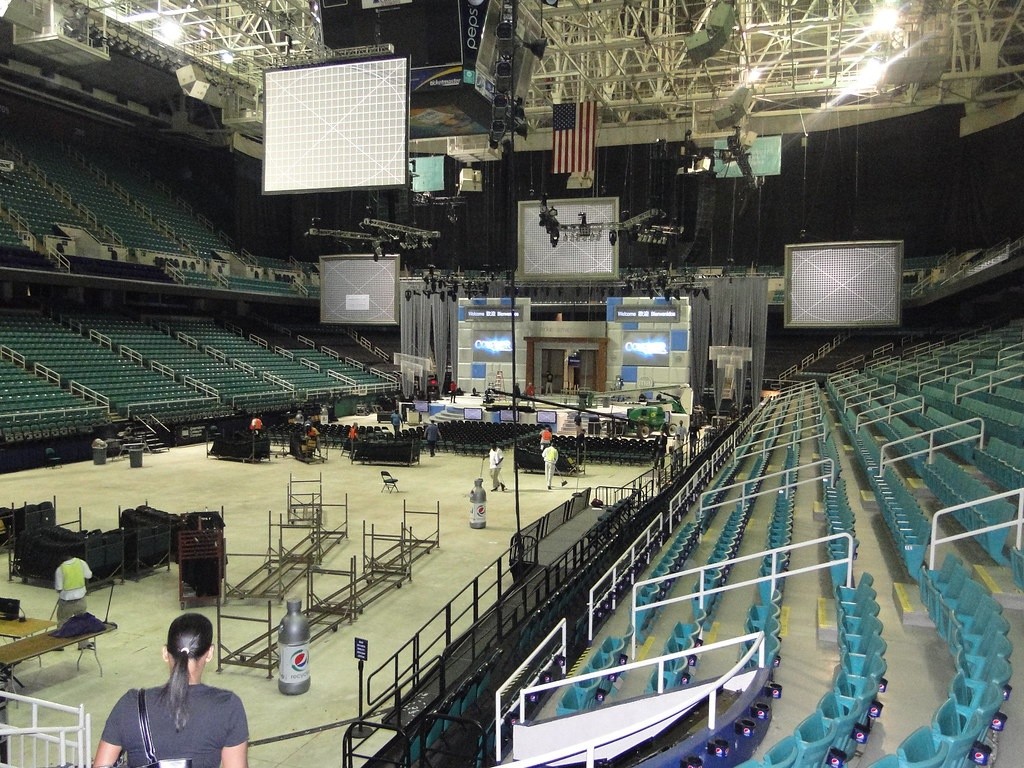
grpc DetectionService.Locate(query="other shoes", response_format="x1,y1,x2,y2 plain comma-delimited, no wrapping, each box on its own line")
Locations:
78,642,95,649
548,486,551,490
52,648,64,651
430,453,434,457
491,488,498,491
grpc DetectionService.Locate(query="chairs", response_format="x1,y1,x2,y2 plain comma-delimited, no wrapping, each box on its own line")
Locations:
0,131,1024,768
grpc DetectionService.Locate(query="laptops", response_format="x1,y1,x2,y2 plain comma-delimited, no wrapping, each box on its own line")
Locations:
0,597,20,621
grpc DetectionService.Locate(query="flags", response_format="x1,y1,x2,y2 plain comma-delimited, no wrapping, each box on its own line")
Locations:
552,101,597,175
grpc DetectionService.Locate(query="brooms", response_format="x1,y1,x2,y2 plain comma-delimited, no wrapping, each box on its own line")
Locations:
553,463,568,487
493,457,506,491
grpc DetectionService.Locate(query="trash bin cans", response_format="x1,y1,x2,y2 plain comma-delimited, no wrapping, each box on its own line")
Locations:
126,443,145,468
90,438,109,466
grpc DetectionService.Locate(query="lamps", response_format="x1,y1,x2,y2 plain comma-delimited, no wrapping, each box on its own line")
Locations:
467,0,561,147
94,26,233,91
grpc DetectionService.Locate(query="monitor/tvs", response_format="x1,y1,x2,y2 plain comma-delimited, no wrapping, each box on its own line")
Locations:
415,401,429,412
463,408,482,420
537,411,557,424
500,410,519,422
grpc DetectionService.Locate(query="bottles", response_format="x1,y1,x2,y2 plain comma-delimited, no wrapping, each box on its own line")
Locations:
469,479,487,529
276,599,312,696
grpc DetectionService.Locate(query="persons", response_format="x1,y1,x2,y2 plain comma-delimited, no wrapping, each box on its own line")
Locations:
544,371,552,396
686,421,700,457
542,440,558,489
390,409,403,432
744,378,801,394
638,391,663,402
672,434,683,469
538,425,551,450
377,380,456,413
52,547,94,651
573,412,581,435
481,440,505,492
249,402,360,459
653,429,667,472
514,382,519,405
525,382,536,408
93,613,249,768
673,420,687,442
424,420,442,457
575,429,585,465
